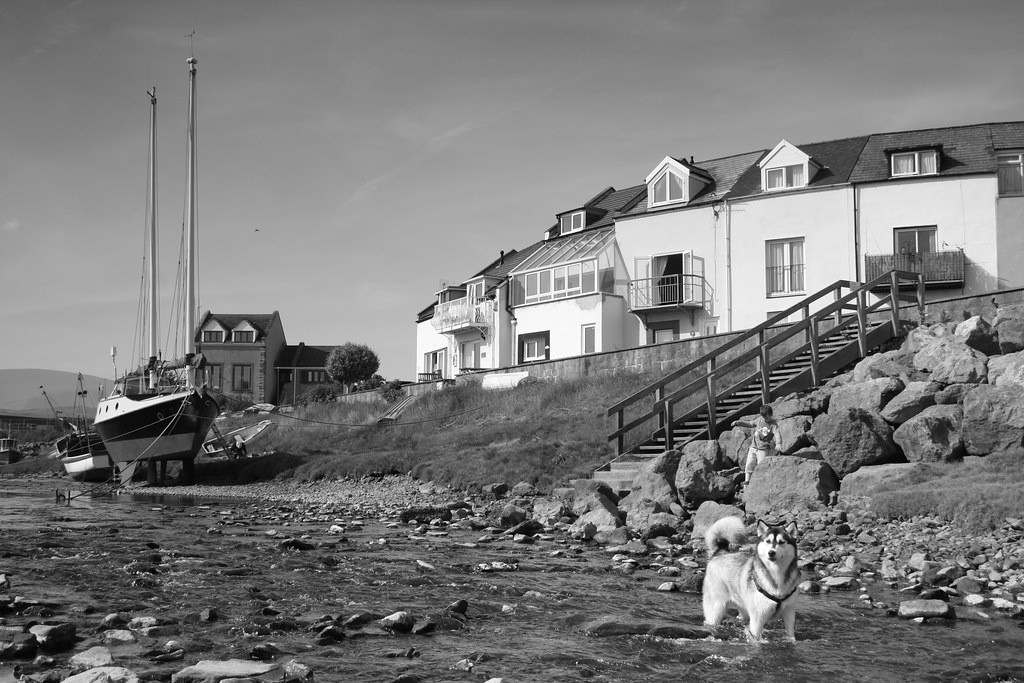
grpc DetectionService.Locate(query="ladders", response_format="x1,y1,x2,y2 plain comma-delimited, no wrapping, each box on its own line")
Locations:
212,424,238,463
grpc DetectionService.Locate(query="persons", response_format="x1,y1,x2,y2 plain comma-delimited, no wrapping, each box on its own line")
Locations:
230,441,247,459
263,442,275,455
731,405,783,485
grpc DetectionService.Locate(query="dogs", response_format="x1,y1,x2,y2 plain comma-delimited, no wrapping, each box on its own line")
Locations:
701,515,801,644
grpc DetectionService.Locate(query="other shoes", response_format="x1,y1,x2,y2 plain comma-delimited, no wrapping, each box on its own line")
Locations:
741,481,749,485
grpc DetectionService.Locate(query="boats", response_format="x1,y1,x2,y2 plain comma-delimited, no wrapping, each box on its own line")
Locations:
202,420,272,455
0,424,23,464
40,372,119,482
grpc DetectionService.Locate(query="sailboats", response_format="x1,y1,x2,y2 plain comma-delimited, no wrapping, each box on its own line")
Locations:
91,29,220,462
